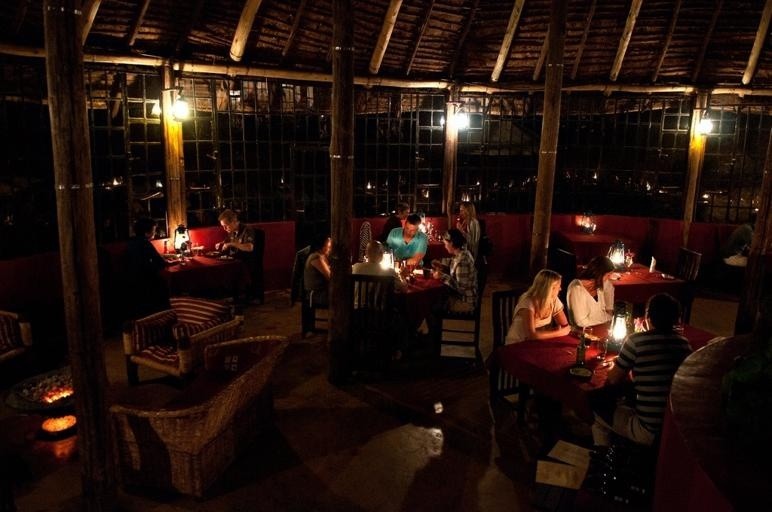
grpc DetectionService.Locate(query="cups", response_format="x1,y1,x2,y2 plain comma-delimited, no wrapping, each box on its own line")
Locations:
393,214,442,279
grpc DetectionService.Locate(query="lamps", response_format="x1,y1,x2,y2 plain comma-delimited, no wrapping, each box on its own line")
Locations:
693,106,713,135
443,99,472,130
160,86,189,120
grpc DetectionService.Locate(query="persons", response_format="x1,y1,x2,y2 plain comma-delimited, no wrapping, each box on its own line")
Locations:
566,254,615,329
582,292,694,492
213,208,257,303
719,212,757,270
126,216,168,310
497,267,601,430
301,199,482,355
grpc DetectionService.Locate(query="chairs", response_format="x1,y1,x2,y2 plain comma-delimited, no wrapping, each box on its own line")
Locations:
714,229,744,302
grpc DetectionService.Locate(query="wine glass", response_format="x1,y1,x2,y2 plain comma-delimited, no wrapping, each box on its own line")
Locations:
582,323,609,364
624,248,633,274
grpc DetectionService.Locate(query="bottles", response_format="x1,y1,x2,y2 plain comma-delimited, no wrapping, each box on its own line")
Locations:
574,332,587,367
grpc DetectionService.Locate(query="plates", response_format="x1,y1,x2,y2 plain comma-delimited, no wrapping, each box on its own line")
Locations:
40,413,78,438
568,367,595,381
